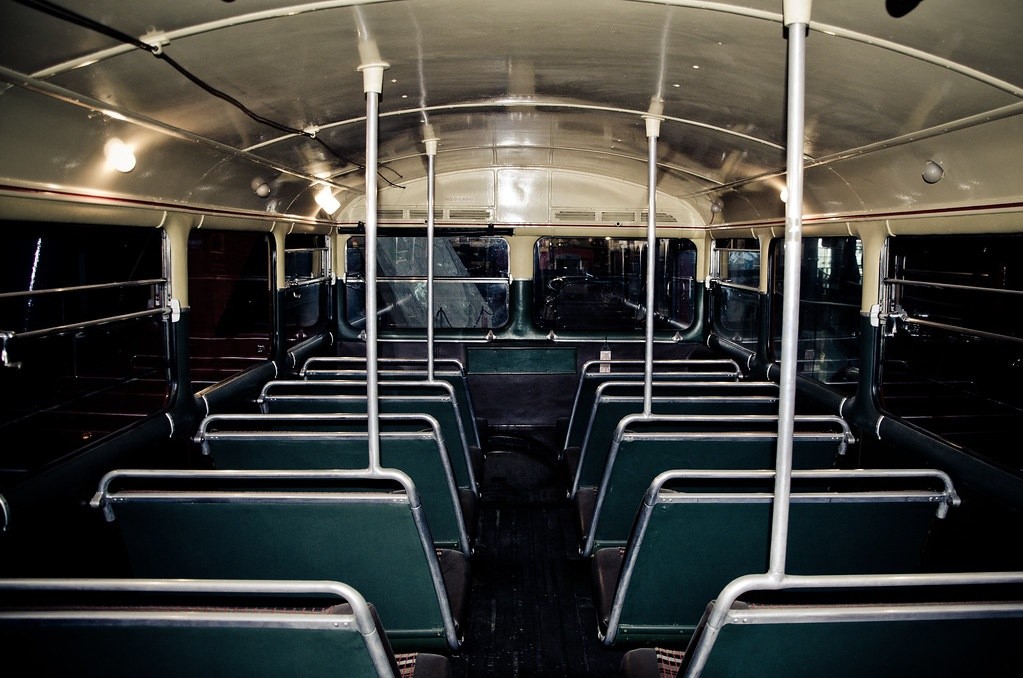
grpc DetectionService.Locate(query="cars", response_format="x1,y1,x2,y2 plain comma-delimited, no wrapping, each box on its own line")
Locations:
0,218,1023,592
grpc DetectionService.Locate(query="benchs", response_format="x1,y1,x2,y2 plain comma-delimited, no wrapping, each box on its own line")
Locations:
588,468,961,652
73,466,471,660
731,333,1023,478
0,335,298,490
250,379,483,513
573,413,856,573
551,359,745,465
289,356,489,468
186,412,484,594
561,380,778,503
0,577,452,678
619,571,1023,678
